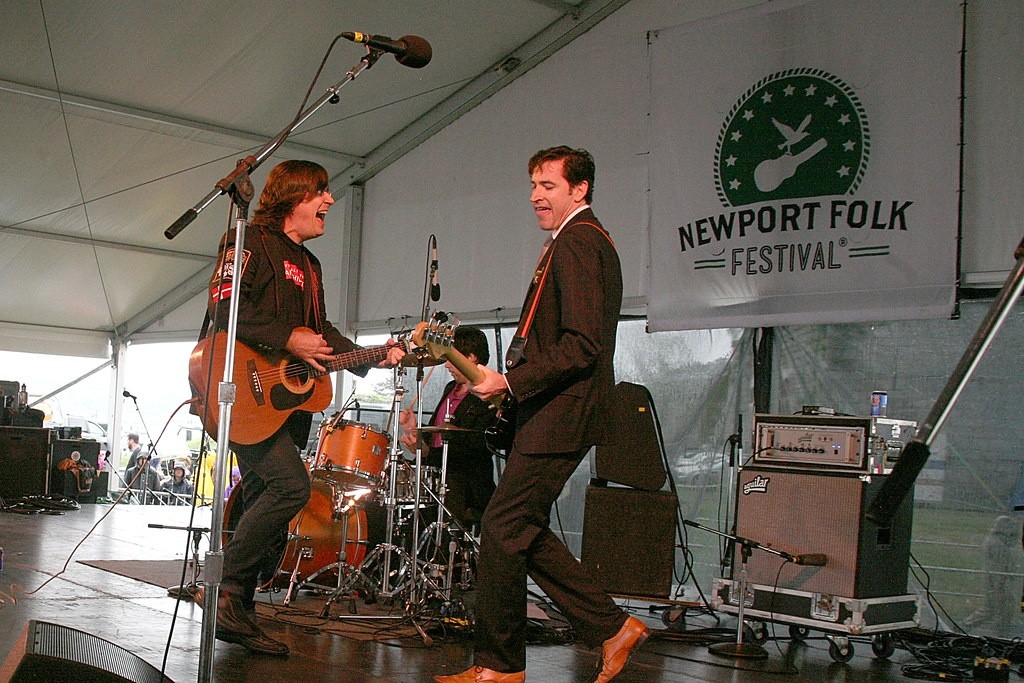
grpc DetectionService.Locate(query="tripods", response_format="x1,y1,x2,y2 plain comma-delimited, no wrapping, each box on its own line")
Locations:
290,348,477,647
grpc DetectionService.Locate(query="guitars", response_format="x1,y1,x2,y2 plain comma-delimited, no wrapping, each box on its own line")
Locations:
187,324,409,457
412,308,527,466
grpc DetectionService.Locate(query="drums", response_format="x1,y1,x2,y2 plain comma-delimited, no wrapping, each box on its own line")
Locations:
309,417,393,490
222,460,369,590
384,463,441,510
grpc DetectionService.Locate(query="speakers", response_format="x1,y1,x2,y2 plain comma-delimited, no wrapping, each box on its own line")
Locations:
729,466,914,599
589,381,668,492
581,484,677,598
0,620,174,683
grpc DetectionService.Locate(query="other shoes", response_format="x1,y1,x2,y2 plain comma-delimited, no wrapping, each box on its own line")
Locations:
194,587,290,656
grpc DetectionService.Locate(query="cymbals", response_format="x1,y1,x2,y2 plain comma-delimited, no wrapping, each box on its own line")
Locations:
399,353,447,368
409,422,478,434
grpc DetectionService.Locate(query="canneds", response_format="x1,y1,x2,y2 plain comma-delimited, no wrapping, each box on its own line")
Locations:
870,390,888,417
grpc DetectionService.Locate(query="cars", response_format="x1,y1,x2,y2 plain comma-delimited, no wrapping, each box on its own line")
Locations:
670,452,729,485
64,415,108,451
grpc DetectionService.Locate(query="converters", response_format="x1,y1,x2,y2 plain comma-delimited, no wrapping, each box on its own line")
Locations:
801,406,835,417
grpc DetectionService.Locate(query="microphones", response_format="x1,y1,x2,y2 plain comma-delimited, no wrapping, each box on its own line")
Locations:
123,391,136,399
341,31,432,68
787,554,828,567
103,450,110,461
430,237,440,301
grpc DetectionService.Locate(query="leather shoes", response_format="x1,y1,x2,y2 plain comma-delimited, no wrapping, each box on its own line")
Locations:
432,664,525,683
593,617,654,683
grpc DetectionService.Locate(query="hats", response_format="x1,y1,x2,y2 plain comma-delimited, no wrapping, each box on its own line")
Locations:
136,452,150,460
173,462,186,469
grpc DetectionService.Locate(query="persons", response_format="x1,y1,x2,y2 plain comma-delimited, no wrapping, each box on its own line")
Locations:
374,325,495,593
124,432,241,505
207,157,407,654
432,145,653,683
